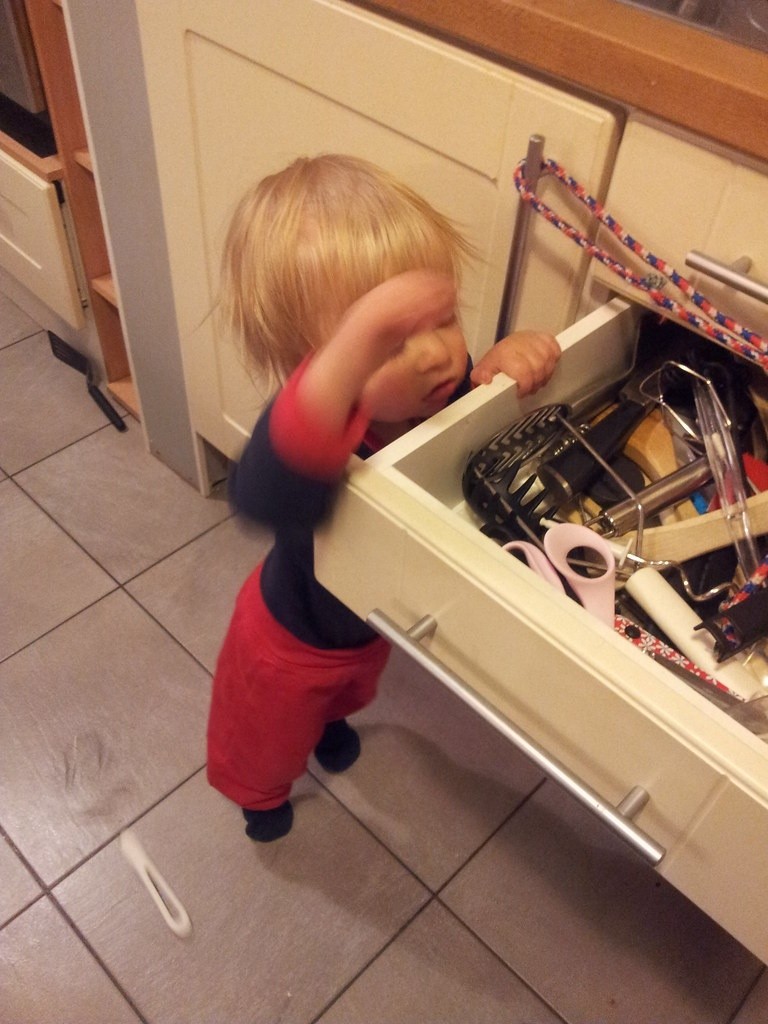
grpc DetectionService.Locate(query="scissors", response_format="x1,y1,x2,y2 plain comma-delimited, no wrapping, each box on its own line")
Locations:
502,520,616,630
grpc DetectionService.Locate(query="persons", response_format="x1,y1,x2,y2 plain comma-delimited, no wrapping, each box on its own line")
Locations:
208,157,562,843
